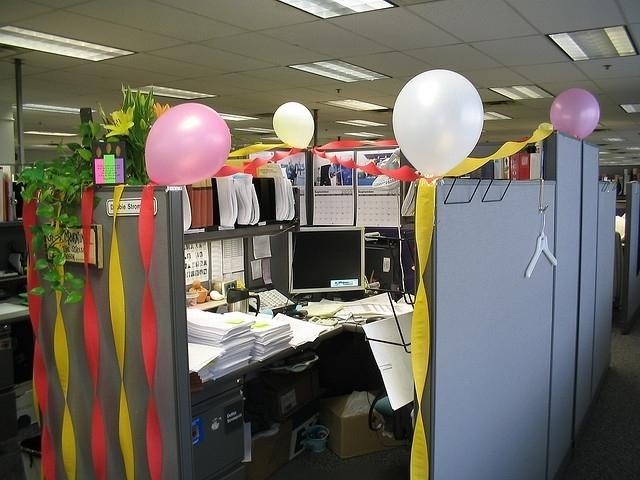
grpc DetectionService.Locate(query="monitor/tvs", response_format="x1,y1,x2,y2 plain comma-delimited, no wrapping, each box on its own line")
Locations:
288,226,365,302
0,221,27,267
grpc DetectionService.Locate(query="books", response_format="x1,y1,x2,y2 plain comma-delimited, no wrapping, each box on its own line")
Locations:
401,180,419,217
0,170,43,223
182,160,297,235
0,302,30,321
185,291,414,393
599,167,640,195
470,142,546,179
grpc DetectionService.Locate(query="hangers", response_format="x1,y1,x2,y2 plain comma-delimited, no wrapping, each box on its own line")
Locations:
522,204,557,280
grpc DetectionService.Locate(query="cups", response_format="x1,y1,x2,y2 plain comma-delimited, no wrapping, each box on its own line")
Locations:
226,287,260,317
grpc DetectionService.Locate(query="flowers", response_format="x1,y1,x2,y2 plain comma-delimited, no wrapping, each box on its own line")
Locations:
100,81,174,187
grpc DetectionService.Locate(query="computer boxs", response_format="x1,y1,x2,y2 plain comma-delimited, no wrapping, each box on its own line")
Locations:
365,228,415,302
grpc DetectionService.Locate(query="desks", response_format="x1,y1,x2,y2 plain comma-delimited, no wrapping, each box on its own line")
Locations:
1,295,38,425
189,297,415,479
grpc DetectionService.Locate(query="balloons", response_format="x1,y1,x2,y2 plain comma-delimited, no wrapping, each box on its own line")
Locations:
272,101,316,151
547,85,602,142
392,68,486,181
143,103,232,187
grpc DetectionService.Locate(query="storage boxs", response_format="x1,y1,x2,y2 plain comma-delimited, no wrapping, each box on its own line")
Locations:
245,367,411,480
